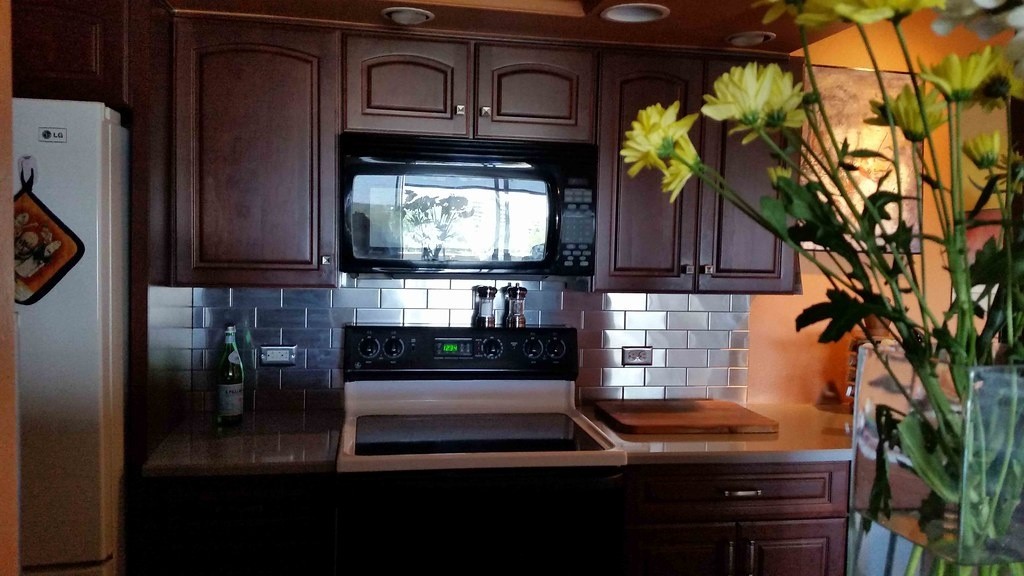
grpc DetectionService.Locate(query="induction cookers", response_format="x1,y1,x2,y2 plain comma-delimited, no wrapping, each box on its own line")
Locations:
335,322,628,468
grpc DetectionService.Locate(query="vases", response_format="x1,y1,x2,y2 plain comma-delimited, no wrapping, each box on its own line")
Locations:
850,339,1023,565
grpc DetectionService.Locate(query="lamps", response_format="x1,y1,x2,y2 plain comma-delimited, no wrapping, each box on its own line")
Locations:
724,30,777,47
381,6,435,26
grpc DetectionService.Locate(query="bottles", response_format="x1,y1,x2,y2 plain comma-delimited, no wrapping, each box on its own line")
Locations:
473,282,530,327
216,325,244,426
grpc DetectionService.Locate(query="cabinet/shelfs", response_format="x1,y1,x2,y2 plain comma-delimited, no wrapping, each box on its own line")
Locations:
590,44,808,295
10,1,341,289
568,422,853,576
341,1,598,144
143,458,344,576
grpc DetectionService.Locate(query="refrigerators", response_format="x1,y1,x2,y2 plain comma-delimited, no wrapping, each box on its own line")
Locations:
13,98,128,576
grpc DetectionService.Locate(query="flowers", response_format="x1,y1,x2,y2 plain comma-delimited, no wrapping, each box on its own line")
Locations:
620,1,1024,576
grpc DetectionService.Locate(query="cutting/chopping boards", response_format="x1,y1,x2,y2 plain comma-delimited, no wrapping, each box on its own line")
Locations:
594,401,782,434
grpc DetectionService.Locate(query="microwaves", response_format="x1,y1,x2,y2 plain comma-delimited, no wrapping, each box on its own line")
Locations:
337,132,597,276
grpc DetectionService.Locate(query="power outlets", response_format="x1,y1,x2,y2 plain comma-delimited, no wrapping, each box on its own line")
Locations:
622,347,653,367
259,345,297,365
